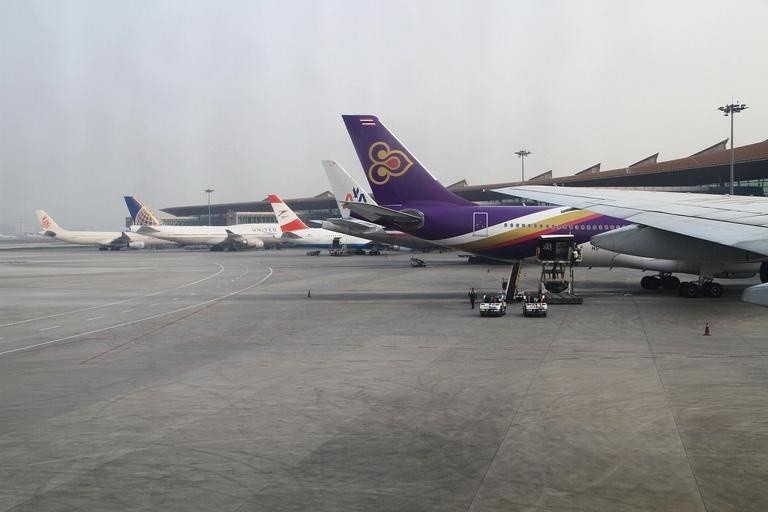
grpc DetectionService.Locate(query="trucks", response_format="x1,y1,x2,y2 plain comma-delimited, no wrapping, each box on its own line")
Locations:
523,295,548,317
479,296,506,316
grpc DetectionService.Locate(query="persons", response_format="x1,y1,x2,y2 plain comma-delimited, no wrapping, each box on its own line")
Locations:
571,250,579,265
468,287,477,309
543,240,552,260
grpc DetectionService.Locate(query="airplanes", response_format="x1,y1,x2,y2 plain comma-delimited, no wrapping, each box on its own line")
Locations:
123,196,283,250
37,210,177,249
269,114,768,306
